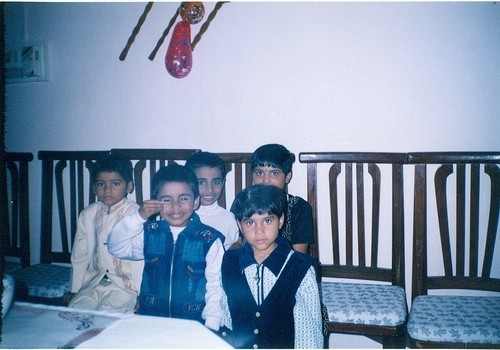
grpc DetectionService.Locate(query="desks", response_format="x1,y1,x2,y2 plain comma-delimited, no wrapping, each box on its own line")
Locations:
0,303,237,349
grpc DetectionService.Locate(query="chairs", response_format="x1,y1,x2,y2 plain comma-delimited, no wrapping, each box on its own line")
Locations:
0,147,500,349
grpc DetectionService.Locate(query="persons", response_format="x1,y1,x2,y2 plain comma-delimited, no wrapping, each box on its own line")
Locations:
183,152,239,250
70,157,145,310
106,164,227,331
229,144,314,254
221,186,327,349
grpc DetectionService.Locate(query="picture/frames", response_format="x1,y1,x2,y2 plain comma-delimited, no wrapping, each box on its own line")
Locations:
5,41,49,84
